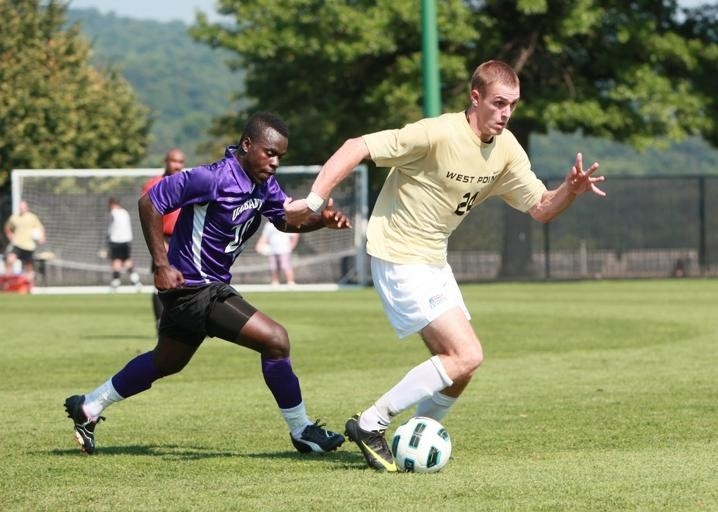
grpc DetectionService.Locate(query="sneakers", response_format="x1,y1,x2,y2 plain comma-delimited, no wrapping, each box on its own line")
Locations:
344,411,399,474
288,418,346,456
62,394,106,456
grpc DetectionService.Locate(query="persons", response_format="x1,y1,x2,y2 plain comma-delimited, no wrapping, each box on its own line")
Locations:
63,110,353,453
96,195,142,288
1,201,47,288
253,217,299,286
279,58,606,472
142,149,188,339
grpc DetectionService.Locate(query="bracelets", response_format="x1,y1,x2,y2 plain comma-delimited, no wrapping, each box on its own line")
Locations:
305,191,325,213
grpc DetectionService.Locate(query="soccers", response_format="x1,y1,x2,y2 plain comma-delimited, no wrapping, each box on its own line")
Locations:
391,417,452,473
